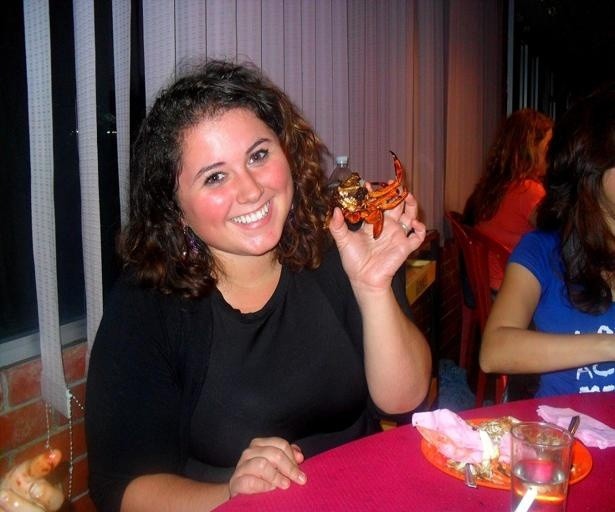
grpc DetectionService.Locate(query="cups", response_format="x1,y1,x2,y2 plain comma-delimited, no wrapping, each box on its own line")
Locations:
510,421,571,511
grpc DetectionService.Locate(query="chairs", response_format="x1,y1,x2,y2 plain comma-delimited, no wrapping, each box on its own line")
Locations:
437,210,510,409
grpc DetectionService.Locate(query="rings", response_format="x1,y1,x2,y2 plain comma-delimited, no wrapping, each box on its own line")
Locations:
398,222,410,233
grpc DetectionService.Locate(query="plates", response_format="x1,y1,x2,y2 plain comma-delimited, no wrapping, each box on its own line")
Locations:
422,418,595,491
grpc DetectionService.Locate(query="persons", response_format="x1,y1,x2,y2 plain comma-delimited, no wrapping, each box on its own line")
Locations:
84,53,442,512
475,103,615,401
457,107,555,404
0,448,68,512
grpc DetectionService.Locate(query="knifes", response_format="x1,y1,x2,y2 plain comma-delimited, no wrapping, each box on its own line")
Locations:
512,414,580,511
